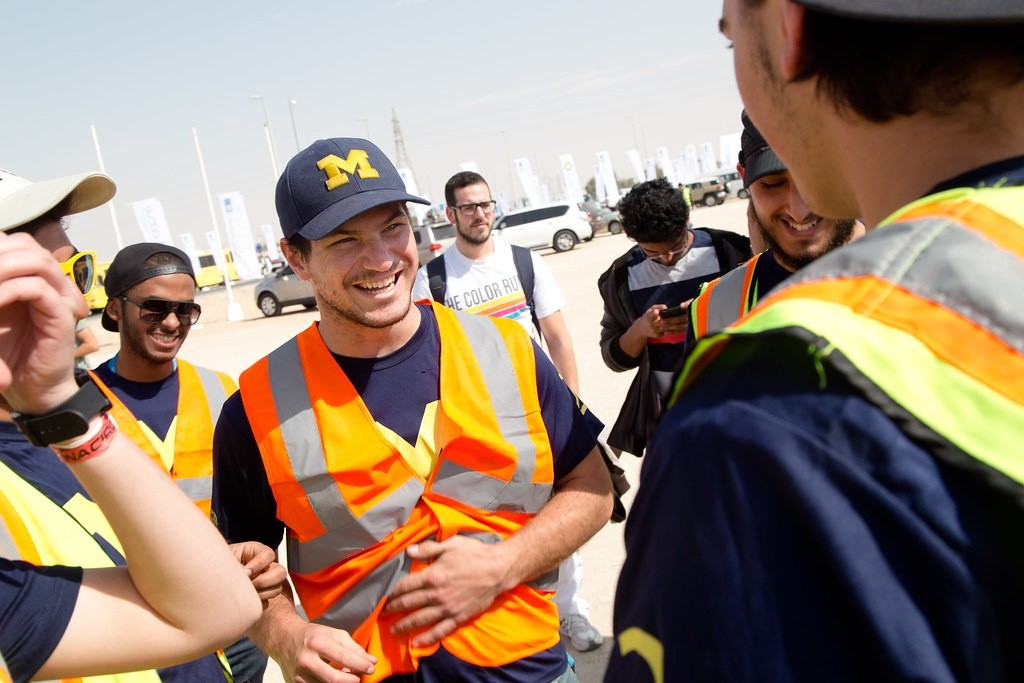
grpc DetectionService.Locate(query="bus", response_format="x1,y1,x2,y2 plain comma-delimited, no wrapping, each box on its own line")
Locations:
84,262,115,312
190,247,239,290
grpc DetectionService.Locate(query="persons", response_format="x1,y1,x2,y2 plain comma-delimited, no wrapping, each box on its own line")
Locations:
408,170,605,653
678,100,868,355
209,137,615,683
597,178,756,460
599,0,1024,683
0,169,286,683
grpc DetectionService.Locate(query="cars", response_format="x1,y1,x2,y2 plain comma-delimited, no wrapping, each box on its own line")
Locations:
577,201,624,241
254,261,317,317
260,258,283,276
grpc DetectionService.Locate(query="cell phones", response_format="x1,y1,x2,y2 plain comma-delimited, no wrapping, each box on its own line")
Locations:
659,305,687,319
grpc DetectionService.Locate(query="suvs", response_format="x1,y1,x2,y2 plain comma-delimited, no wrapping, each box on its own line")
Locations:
684,178,726,206
717,172,750,199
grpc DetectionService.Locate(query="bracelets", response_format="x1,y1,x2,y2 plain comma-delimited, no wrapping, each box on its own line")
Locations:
47,411,119,464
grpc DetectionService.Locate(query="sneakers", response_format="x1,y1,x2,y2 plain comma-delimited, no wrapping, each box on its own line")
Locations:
558,614,604,652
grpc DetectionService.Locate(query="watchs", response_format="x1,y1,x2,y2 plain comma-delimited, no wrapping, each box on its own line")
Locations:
10,366,113,448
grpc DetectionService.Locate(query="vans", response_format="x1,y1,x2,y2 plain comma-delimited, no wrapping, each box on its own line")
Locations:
413,220,457,268
488,201,593,253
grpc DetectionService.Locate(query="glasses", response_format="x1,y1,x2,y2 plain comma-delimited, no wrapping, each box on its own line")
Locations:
59,250,97,296
638,225,687,259
119,294,201,326
451,200,497,216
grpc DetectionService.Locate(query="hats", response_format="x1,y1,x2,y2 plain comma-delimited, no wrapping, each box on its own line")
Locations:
0,167,118,231
741,107,788,188
275,138,432,242
101,242,197,332
